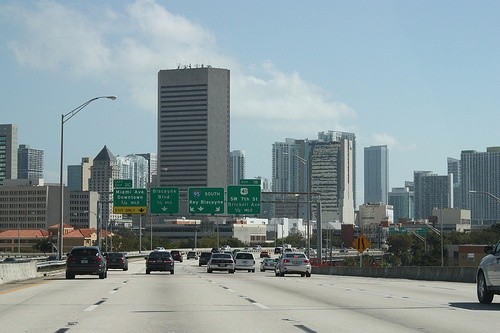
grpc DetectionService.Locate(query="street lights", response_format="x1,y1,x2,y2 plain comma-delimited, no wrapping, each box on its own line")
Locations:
416,192,444,266
468,190,500,202
59,95,117,259
281,151,310,259
139,167,168,253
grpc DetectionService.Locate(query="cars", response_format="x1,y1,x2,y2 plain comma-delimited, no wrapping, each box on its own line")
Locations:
234,252,256,273
274,252,312,277
211,247,220,253
185,252,198,260
106,253,128,271
199,252,212,266
171,251,183,262
221,243,348,259
260,258,277,272
206,253,234,274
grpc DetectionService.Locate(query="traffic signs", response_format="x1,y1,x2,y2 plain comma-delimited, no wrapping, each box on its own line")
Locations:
188,186,224,214
149,187,180,214
112,188,148,214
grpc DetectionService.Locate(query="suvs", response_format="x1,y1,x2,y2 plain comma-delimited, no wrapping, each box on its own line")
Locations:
144,251,174,275
65,246,108,279
